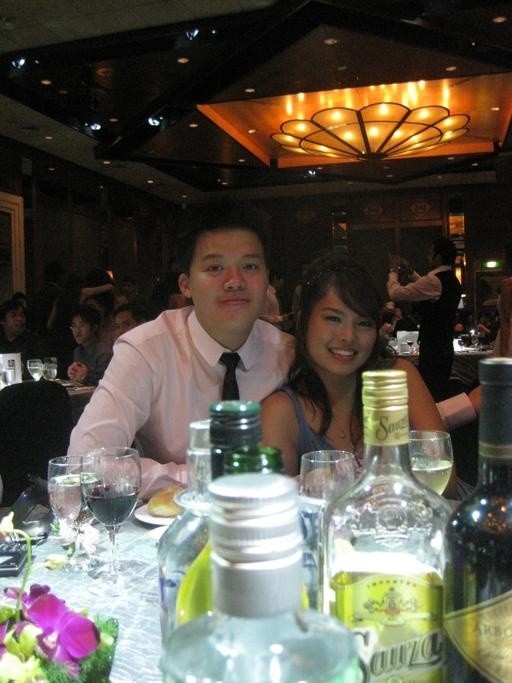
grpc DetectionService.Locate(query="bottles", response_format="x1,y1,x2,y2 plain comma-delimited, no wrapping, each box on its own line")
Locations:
440,353,511,682
155,418,213,646
173,397,309,648
156,468,365,683
256,442,286,476
318,368,460,682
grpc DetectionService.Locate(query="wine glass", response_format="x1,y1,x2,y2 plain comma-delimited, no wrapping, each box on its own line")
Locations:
28,356,59,381
476,331,486,347
47,444,143,597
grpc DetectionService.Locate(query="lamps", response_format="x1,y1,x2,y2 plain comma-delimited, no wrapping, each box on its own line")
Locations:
271,80,472,162
145,103,169,131
6,54,29,71
179,21,203,39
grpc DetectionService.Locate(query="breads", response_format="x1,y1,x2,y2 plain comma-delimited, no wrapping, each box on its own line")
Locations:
148,486,184,517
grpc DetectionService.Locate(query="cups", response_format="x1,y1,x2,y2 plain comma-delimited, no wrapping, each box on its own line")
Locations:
460,334,469,345
301,451,359,508
408,426,453,494
1,365,17,386
397,330,419,354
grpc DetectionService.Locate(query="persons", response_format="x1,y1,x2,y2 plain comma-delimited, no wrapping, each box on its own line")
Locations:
1,260,191,387
61,213,482,503
493,277,512,357
258,249,458,501
380,302,419,339
477,311,500,344
385,236,463,405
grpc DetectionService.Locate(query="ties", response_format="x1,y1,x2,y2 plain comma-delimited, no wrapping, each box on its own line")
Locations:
220,353,241,402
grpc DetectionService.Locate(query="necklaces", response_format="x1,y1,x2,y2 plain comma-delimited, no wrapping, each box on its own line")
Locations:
317,406,360,439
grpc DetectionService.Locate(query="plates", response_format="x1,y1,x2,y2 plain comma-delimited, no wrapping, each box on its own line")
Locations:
132,502,174,526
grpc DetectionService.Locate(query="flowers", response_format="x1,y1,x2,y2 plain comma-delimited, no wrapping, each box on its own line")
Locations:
0,584,120,681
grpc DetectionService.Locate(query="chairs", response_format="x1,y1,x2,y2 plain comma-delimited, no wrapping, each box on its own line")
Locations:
1,382,71,504
